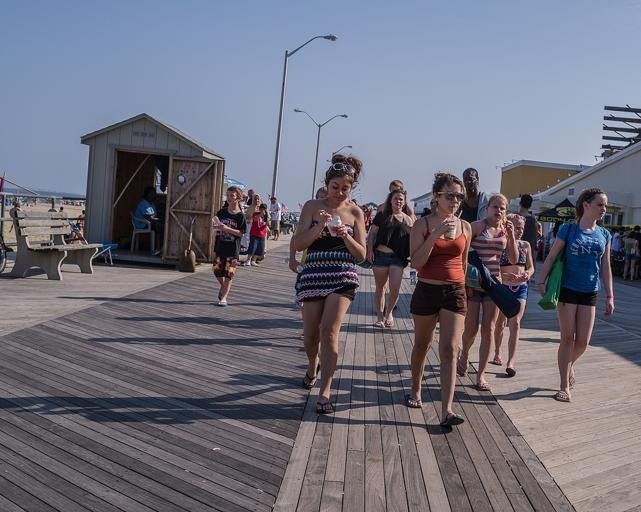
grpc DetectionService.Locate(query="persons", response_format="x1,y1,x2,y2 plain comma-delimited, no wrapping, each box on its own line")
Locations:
613,223,641,277
213,186,247,306
8,202,26,234
349,179,417,327
12,199,18,206
316,187,328,199
455,168,490,223
537,184,614,402
242,183,296,266
59,206,68,222
456,193,536,391
293,153,366,415
133,186,166,255
406,173,472,426
79,210,87,233
516,194,542,283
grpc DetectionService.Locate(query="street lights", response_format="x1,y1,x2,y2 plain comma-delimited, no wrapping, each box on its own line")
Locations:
268,33,338,201
331,144,352,156
292,107,348,202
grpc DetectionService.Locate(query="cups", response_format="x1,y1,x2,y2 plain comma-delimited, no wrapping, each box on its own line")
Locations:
327,216,343,237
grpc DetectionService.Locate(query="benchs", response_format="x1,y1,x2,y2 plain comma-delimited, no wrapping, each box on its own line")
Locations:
8,207,101,279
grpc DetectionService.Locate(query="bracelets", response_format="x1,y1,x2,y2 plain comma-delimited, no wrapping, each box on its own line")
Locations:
538,283,544,285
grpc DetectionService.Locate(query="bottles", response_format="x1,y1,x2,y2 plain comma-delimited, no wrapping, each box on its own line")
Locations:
212,216,220,226
410,265,418,286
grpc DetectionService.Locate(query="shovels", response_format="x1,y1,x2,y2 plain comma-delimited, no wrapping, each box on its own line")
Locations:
179,214,198,272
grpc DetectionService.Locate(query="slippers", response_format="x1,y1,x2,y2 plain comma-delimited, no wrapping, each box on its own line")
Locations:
303,363,320,390
494,357,503,366
316,401,334,414
217,301,227,307
506,367,517,377
439,412,465,426
556,390,570,401
406,394,422,408
457,363,469,377
477,382,492,392
376,319,394,327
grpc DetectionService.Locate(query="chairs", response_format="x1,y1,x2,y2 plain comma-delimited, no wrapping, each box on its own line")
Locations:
128,212,157,253
68,222,118,266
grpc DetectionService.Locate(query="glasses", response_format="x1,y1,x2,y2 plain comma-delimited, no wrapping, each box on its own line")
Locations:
438,191,465,201
328,163,355,174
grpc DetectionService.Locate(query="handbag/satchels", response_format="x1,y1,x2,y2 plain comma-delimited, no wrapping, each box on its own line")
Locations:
354,258,372,269
